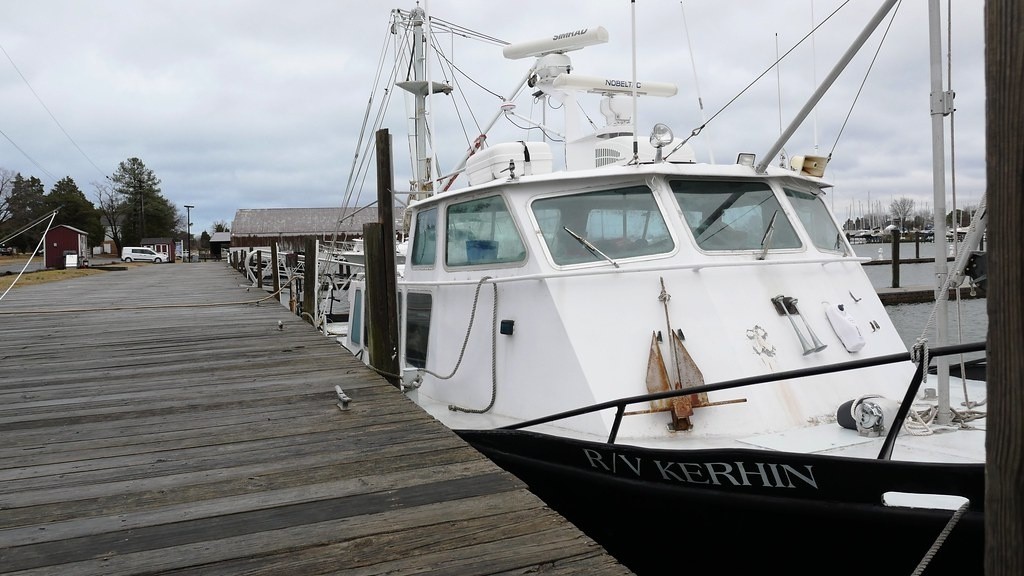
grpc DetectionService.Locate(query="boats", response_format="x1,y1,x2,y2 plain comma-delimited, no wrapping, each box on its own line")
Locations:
345,1,987,510
324,222,559,276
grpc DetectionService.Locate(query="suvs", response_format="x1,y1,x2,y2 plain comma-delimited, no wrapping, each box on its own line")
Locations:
120,247,169,263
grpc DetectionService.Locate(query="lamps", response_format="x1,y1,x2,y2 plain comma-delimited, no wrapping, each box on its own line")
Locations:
649,123,674,148
736,153,756,165
